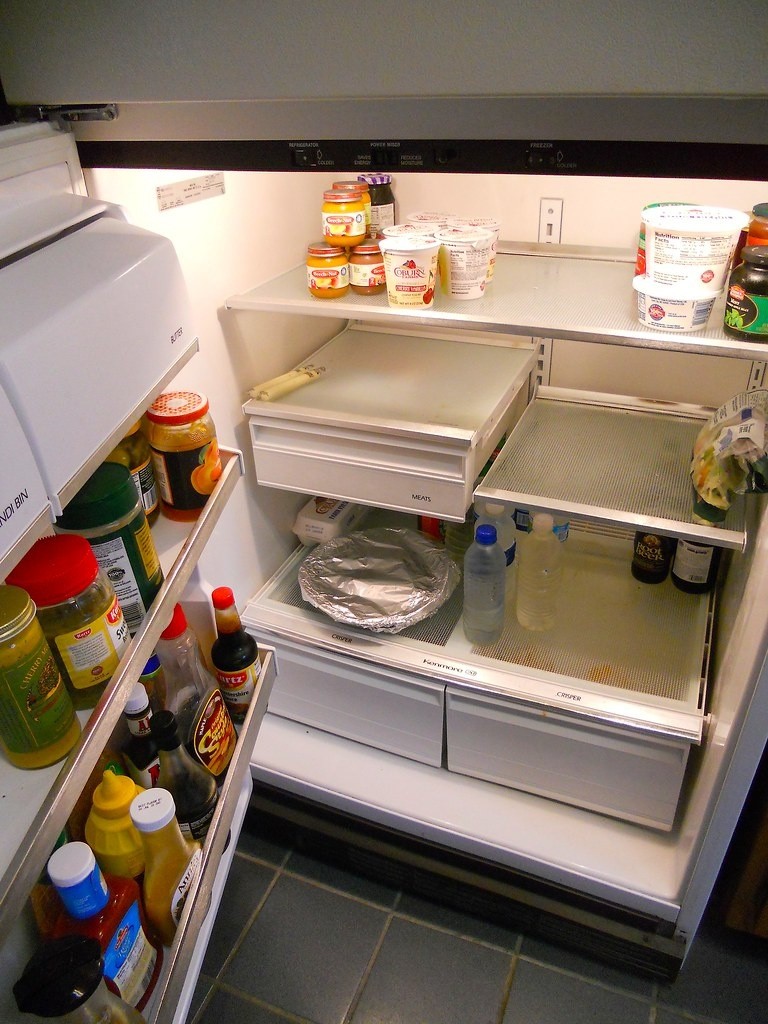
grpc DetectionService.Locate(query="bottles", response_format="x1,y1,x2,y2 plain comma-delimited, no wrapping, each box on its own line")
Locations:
512,507,534,574
552,512,570,544
129,790,203,949
632,529,678,585
13,935,149,1024
421,516,445,551
516,512,563,632
150,705,220,850
209,586,262,724
84,770,148,892
462,523,505,644
670,535,720,593
124,682,163,788
444,509,474,586
474,502,516,606
475,499,516,522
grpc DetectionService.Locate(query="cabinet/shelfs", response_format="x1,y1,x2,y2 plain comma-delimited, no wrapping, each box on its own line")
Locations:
0,447,280,1023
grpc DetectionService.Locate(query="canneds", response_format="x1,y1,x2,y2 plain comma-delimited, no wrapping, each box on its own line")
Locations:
720,202,768,345
0,390,223,770
305,173,398,298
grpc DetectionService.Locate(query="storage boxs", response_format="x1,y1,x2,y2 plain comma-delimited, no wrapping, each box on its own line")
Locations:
240,619,711,833
244,329,542,523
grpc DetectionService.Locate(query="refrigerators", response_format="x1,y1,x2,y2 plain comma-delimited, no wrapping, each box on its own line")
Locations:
0,0,768,1024
